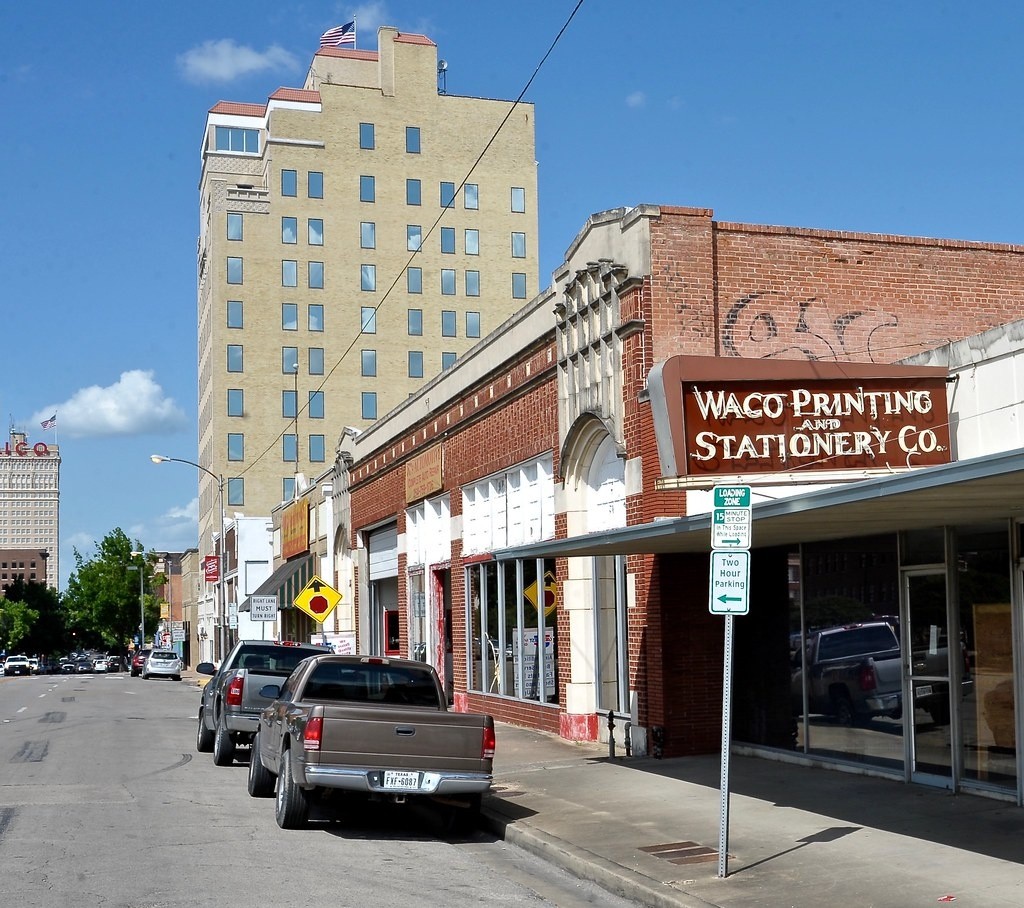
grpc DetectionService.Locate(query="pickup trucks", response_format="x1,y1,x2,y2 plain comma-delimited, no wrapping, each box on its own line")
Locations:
190,641,338,768
792,624,957,728
244,654,492,835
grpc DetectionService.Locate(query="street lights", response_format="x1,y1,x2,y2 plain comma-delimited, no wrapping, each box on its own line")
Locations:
128,564,146,651
150,454,227,664
132,549,173,651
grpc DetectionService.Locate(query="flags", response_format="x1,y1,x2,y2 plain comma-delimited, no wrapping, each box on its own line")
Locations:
40,414,57,431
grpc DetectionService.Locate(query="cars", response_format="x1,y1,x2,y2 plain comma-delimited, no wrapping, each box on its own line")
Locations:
131,648,150,675
144,650,182,682
0,656,40,674
57,656,124,673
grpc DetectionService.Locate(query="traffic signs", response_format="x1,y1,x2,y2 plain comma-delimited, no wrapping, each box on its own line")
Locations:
709,484,752,617
